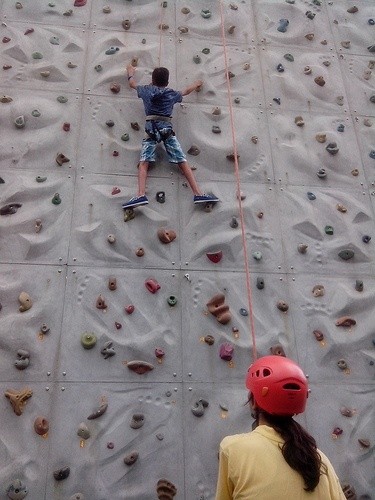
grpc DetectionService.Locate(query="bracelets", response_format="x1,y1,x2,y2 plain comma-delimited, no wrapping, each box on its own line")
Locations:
128,75,135,80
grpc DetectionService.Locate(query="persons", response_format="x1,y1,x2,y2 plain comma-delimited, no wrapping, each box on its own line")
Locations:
121,63,222,211
214,356,350,500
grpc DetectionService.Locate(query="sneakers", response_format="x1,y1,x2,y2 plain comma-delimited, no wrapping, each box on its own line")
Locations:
121,194,149,209
194,193,220,204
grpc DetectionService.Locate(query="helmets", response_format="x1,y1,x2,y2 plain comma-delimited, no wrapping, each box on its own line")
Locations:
245,355,308,414
